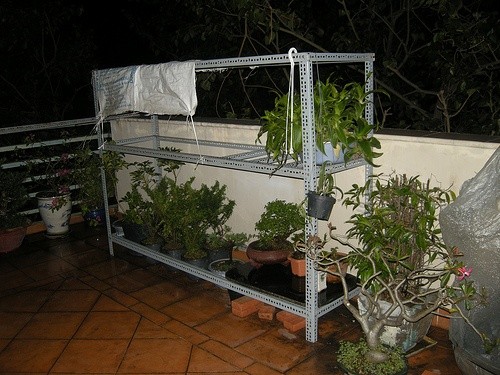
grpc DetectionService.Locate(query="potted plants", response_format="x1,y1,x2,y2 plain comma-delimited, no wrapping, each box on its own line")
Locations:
0,155,32,253
341,171,456,352
76,142,109,228
254,71,391,178
97,139,349,309
307,160,343,221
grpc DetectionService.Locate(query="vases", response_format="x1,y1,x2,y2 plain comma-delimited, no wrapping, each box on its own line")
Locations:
337,340,410,375
35,192,72,235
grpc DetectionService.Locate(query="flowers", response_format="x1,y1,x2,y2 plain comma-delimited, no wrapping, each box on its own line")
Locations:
296,223,500,375
15,130,77,212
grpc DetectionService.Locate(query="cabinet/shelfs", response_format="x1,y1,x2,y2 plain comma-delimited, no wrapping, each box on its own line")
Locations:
92,52,375,343
